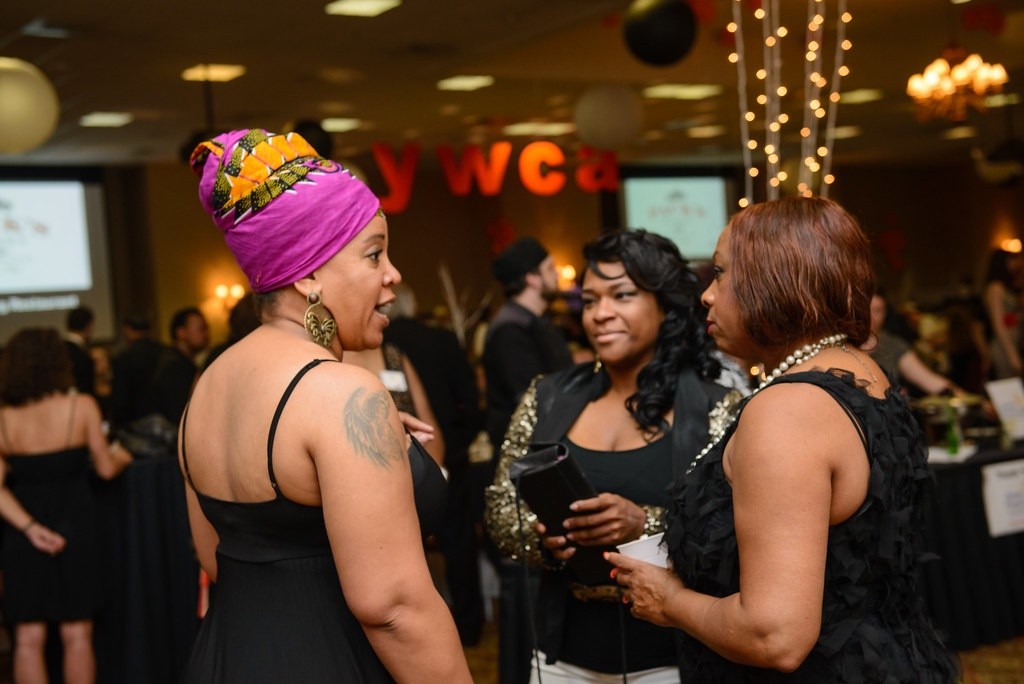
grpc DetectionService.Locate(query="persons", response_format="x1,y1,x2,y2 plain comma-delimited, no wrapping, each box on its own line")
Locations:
484,224,743,684
601,196,960,683
0,235,561,683
861,285,978,401
969,245,1024,395
178,128,474,684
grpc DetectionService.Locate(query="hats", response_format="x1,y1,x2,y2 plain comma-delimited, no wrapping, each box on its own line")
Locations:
490,235,548,283
191,128,381,292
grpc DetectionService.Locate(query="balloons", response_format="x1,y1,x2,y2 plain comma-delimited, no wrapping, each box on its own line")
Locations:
0,55,59,160
621,0,700,68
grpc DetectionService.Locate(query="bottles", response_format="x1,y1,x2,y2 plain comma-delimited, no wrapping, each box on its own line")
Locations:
946,418,958,453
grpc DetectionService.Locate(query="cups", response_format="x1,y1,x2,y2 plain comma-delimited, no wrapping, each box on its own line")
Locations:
616,532,672,571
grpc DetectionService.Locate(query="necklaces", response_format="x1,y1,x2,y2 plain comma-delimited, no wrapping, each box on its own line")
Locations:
686,331,878,473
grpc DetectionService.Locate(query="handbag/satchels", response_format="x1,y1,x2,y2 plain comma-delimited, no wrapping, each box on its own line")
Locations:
509,439,619,580
